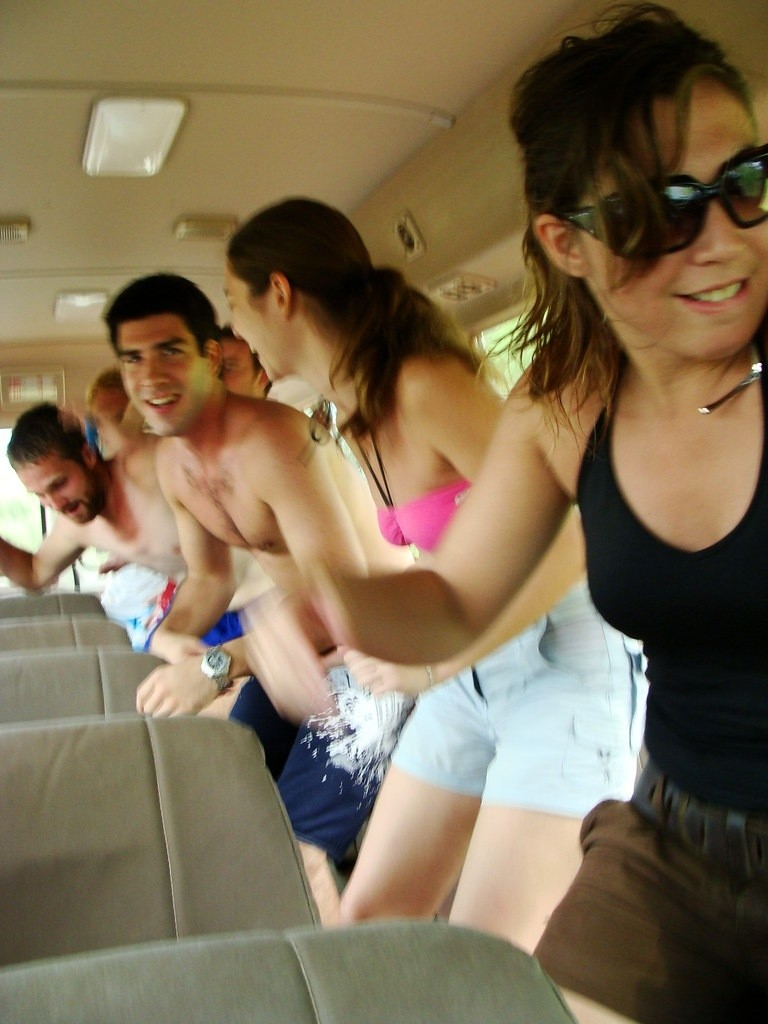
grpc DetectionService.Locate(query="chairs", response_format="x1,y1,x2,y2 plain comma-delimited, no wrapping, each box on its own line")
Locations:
0,595,581,1024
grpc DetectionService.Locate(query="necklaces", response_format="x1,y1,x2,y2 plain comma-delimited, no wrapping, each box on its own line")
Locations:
697,356,766,415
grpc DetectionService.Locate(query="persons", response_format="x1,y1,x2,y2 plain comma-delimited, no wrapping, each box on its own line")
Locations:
0,196,650,956
247,0,767,1024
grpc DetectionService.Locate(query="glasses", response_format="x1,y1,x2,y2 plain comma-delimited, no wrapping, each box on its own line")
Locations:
554,142,768,260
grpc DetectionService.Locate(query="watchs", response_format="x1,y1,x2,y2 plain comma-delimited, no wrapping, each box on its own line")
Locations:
201,645,233,693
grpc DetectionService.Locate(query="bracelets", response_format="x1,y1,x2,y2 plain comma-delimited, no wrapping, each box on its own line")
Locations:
424,666,433,688
